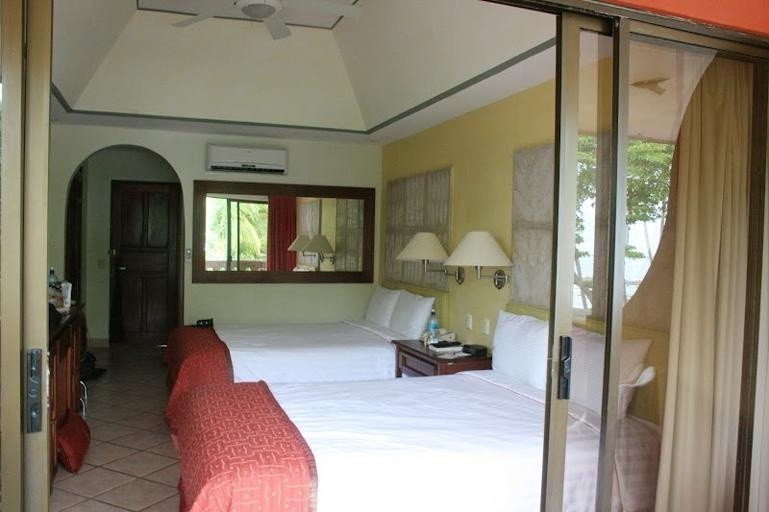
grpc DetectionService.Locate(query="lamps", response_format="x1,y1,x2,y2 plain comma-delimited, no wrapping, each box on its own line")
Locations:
302,230,338,265
439,224,510,290
391,229,468,287
286,227,323,263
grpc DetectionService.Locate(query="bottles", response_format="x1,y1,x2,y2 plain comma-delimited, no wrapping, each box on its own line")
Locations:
48,265,65,309
426,308,439,343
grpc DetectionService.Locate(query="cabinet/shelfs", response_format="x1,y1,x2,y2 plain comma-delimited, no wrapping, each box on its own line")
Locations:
46,299,93,486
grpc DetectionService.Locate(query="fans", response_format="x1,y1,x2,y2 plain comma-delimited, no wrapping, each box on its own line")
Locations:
170,0,363,43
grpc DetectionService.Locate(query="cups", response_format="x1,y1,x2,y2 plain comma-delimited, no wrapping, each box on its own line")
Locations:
61,282,72,308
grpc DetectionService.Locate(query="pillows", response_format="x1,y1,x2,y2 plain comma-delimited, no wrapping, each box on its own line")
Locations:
387,288,437,348
364,282,400,331
541,324,652,420
487,307,553,391
55,405,92,474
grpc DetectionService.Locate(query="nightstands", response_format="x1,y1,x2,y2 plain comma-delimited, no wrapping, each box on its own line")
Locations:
391,333,489,378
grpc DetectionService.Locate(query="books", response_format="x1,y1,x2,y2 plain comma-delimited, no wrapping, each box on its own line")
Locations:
427,343,463,352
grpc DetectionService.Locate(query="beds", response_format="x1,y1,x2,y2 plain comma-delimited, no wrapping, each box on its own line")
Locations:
165,369,659,512
160,321,395,422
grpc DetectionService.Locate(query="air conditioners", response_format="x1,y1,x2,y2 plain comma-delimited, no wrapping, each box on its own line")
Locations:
202,141,291,180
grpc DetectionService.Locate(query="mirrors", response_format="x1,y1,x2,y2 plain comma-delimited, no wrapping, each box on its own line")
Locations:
189,176,377,287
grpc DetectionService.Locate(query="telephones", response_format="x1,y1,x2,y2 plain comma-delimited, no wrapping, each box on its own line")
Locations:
420,328,457,344
